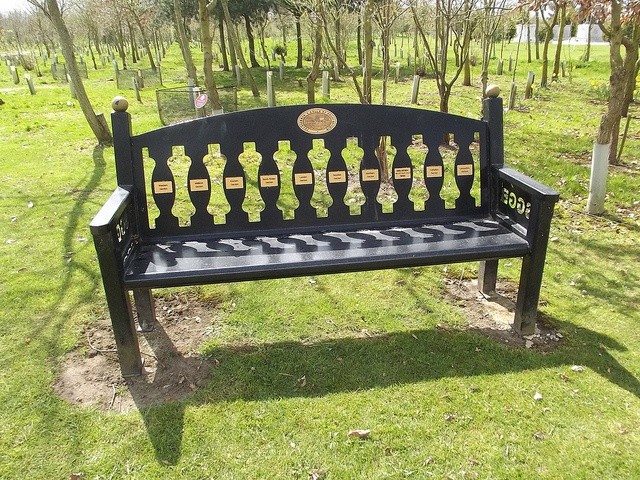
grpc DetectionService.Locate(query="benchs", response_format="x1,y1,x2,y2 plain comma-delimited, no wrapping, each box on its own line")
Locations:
89,83,560,378
